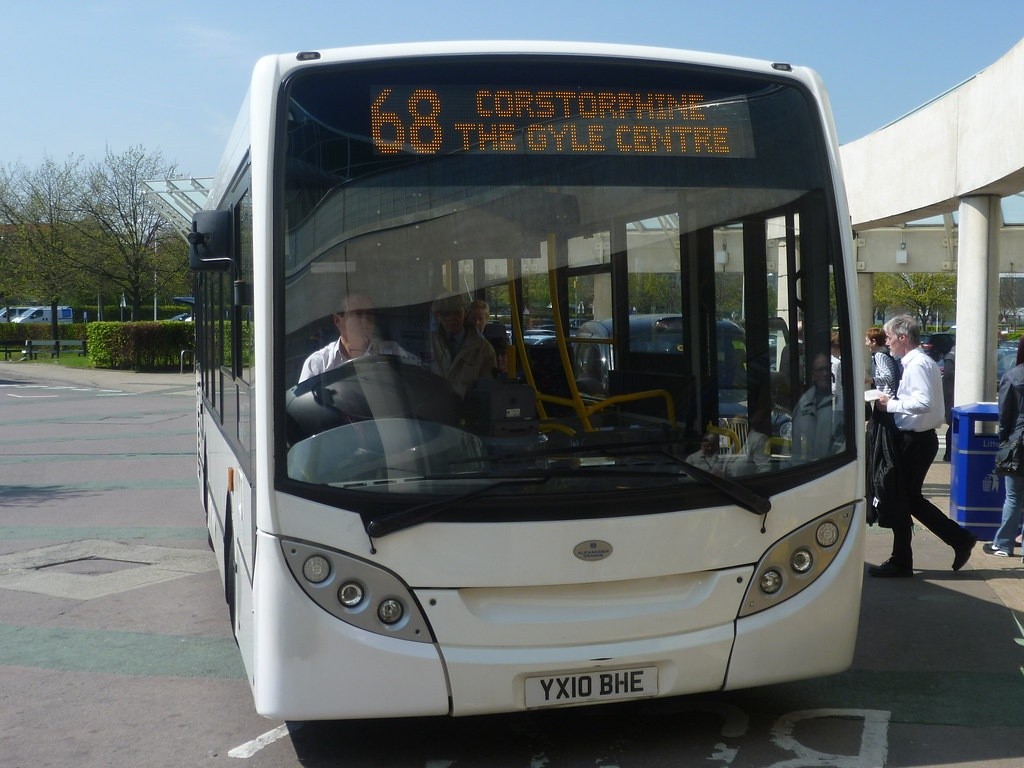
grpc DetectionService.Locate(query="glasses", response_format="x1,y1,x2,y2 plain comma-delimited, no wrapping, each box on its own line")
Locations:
812,364,830,374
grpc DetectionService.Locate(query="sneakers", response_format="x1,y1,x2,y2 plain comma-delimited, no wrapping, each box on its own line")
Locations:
868,558,913,577
983,543,1011,559
951,534,976,572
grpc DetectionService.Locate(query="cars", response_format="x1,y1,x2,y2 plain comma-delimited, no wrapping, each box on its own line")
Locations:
503,314,794,440
919,330,1020,423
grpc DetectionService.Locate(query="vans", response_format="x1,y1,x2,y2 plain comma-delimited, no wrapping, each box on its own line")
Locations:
0,306,32,322
11,306,73,324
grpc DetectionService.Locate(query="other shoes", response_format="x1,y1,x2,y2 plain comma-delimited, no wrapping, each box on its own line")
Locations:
1015,535,1021,547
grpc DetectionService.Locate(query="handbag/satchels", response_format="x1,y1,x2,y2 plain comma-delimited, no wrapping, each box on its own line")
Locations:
995,435,1023,475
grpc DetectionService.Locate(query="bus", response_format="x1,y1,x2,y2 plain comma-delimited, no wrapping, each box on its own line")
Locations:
189,40,868,722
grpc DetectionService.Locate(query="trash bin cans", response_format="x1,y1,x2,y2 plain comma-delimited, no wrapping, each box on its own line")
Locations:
951,401,1024,542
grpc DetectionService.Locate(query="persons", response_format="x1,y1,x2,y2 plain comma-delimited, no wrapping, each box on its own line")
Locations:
780,314,1024,578
298,281,511,408
673,351,846,485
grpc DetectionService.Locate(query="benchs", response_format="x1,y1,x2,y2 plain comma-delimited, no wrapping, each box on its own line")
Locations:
0,340,87,361
